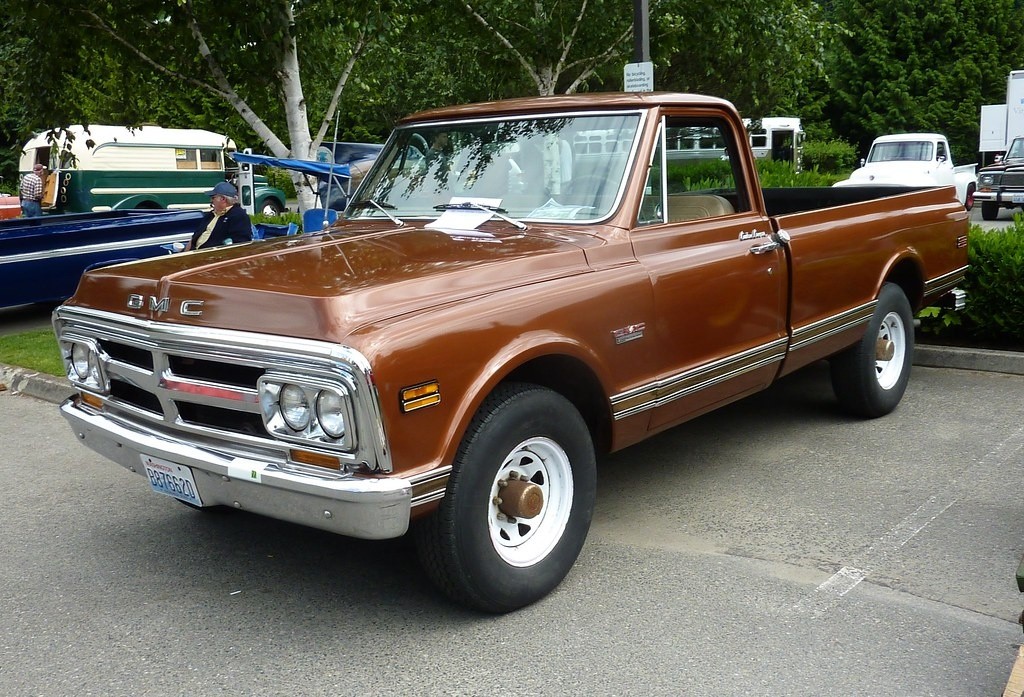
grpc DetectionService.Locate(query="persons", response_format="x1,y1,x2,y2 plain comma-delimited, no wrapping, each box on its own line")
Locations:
558,134,573,192
19,164,47,217
425,132,455,196
180,182,252,252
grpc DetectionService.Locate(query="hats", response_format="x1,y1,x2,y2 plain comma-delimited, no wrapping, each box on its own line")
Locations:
33,164,47,171
205,182,238,198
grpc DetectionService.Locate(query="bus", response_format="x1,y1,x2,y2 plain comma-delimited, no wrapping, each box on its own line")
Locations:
19,124,292,224
571,114,804,170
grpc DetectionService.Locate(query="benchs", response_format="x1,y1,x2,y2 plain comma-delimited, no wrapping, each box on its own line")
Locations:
497,194,736,222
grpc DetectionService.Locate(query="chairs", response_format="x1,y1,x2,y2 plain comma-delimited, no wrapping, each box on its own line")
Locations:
160,222,297,255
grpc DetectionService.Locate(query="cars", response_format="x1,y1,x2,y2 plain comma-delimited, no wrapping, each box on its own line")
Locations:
314,141,433,210
972,135,1024,220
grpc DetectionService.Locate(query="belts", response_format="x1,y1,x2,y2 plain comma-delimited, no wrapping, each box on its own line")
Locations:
23,198,39,202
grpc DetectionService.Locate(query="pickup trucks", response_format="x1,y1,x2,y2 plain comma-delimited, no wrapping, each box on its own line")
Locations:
832,133,979,211
0,209,204,313
51,95,970,620
